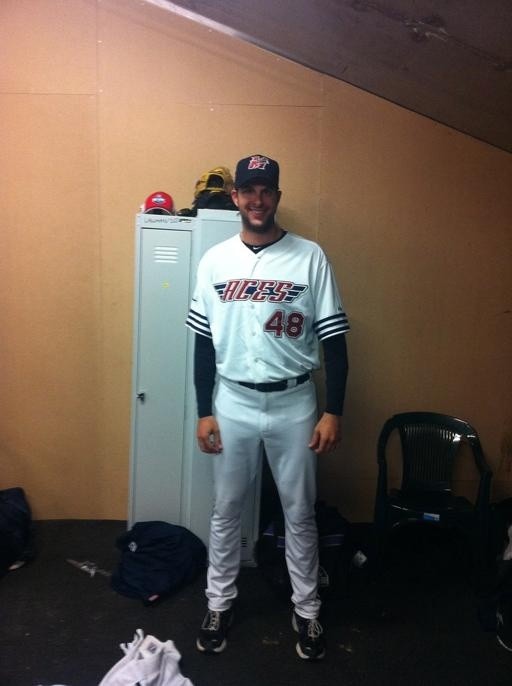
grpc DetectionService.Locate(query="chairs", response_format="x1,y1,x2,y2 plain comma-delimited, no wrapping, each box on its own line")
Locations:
292,611,325,660
196,607,234,653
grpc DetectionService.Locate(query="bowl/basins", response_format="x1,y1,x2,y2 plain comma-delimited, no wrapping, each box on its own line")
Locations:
274,224,278,241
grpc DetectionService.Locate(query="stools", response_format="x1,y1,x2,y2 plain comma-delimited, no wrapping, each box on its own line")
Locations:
238,372,311,393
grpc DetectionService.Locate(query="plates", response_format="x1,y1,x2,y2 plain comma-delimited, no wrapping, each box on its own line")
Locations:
126,209,194,541
188,205,261,569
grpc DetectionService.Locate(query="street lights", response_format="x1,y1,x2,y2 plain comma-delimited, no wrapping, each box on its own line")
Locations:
109,520,209,606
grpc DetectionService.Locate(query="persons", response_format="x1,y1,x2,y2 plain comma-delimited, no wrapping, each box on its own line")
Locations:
184,153,351,660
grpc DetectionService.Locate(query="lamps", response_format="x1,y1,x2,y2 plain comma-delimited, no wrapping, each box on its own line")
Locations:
375,412,494,572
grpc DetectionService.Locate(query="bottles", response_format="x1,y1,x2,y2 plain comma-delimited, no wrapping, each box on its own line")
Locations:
145,192,173,214
194,165,232,199
235,154,280,190
